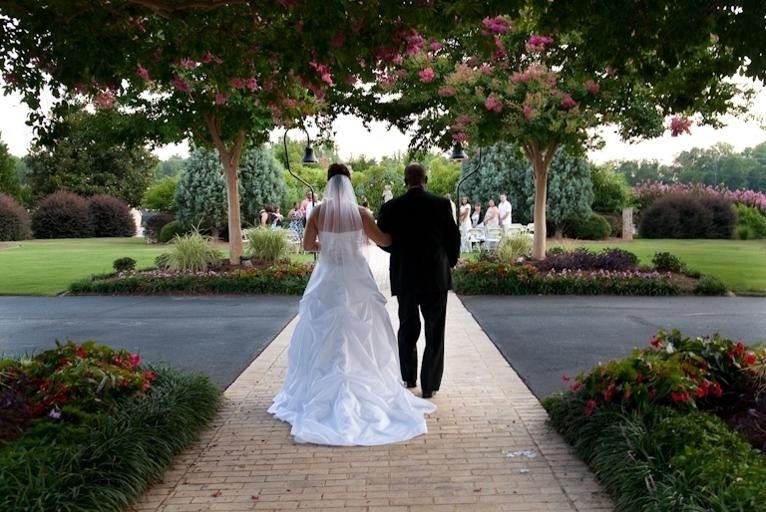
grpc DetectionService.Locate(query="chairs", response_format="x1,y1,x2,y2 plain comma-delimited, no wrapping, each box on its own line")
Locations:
459,222,534,253
239,228,301,254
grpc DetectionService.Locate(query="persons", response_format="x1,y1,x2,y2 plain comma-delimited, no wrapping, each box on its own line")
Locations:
303,162,462,399
258,185,512,252
